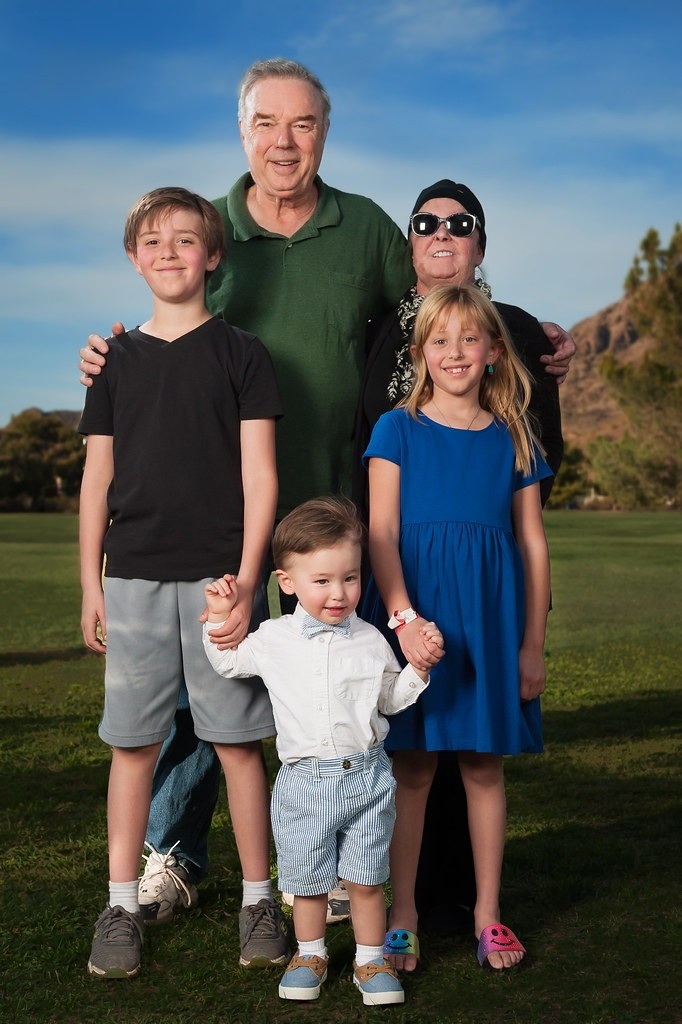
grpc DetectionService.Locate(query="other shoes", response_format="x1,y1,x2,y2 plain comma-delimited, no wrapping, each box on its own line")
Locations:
424,900,471,935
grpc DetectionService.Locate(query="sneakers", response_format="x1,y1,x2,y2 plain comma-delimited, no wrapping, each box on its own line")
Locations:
282,882,352,923
279,950,329,1000
87,902,145,978
138,838,198,925
238,900,292,966
352,957,405,1005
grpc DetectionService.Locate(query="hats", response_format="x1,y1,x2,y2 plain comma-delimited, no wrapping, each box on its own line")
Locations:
408,179,487,258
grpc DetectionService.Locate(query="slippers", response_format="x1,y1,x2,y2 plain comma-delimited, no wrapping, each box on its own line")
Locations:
383,904,423,974
469,923,527,971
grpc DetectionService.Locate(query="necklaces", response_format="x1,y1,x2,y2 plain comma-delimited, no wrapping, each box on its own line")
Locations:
431,399,480,430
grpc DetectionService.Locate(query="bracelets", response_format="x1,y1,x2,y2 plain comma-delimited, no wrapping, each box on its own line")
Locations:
387,606,420,636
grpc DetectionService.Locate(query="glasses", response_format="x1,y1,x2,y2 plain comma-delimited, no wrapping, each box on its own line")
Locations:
409,212,481,238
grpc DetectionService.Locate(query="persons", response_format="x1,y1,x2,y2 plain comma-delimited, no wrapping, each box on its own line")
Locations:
76,57,577,1005
